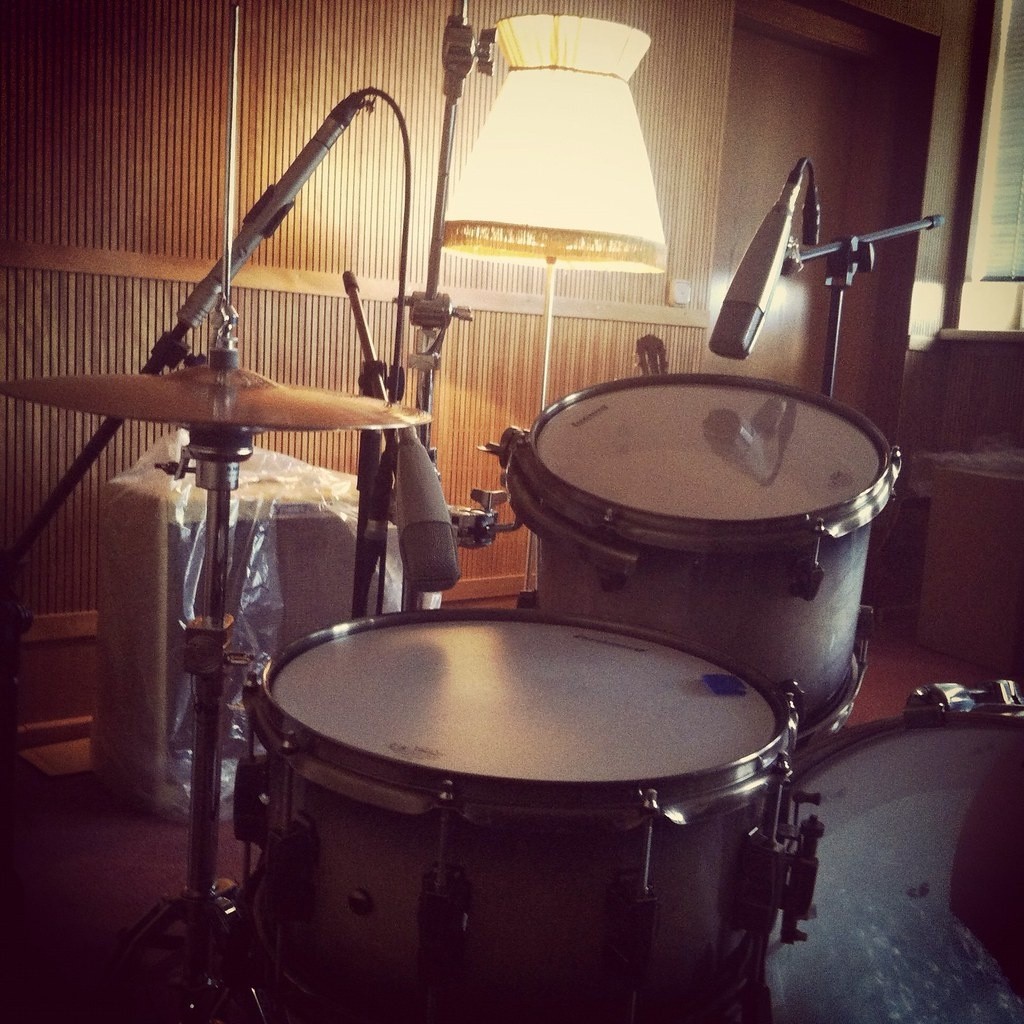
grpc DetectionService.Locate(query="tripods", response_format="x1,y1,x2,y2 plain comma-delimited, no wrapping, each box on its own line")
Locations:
58,424,281,1024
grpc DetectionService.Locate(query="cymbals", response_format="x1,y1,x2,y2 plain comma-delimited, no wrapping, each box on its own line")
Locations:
0,365,436,431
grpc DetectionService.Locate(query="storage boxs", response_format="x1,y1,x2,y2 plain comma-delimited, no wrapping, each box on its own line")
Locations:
88,462,442,825
914,466,1024,675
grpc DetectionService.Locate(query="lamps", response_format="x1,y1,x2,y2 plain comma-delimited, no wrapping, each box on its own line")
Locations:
442,11,671,609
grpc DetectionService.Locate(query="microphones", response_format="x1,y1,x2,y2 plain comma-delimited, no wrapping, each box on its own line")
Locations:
710,169,806,360
396,426,464,592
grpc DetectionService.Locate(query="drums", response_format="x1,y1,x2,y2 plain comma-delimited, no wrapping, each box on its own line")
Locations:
749,681,1023,1023
234,606,825,1023
497,373,907,755
